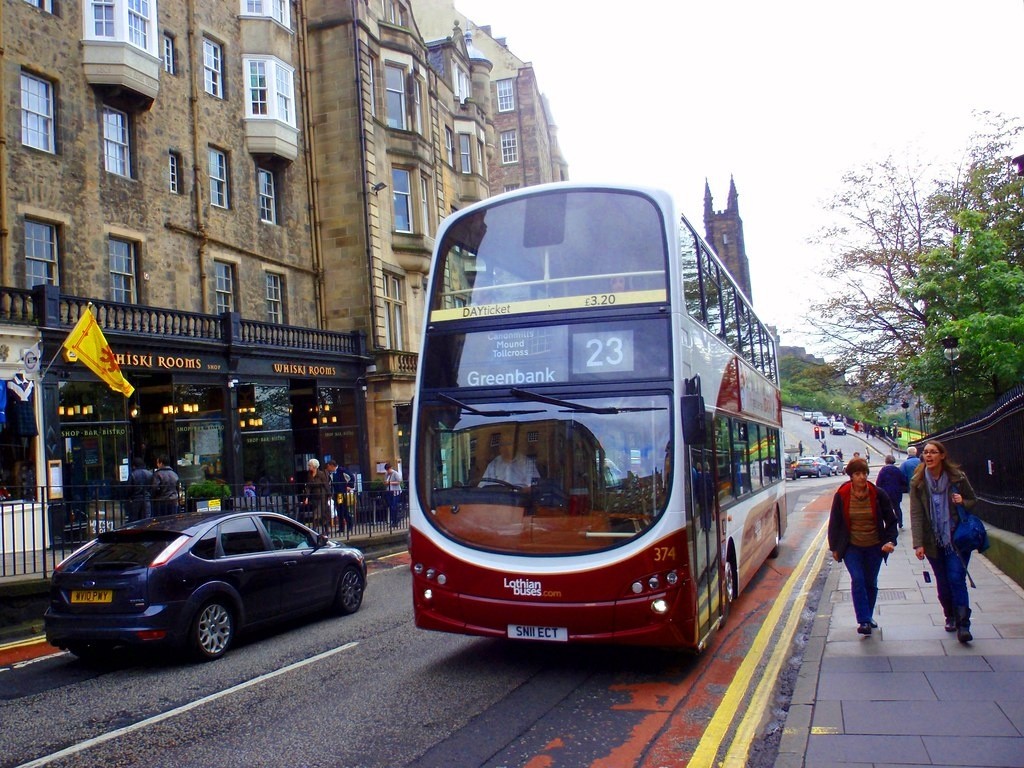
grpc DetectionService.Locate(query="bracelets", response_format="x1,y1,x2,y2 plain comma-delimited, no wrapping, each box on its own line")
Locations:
889,541,894,545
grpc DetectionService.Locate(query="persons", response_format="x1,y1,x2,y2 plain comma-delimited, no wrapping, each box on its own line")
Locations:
610,276,632,292
854,452,860,457
854,420,882,439
910,440,978,641
8,374,37,437
129,454,184,520
832,413,847,426
244,482,256,510
900,448,921,528
799,441,802,456
875,455,905,528
829,458,899,635
477,432,541,494
384,465,402,527
305,458,355,533
821,439,843,460
690,457,776,531
468,282,494,305
865,447,871,463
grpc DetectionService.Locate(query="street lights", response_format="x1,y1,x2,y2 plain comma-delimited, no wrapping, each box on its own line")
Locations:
941,335,961,426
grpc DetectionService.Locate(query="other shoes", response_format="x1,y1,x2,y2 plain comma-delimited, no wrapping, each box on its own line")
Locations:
945,618,958,631
857,621,872,635
958,629,973,643
348,524,353,531
899,524,903,528
337,529,343,532
870,619,877,627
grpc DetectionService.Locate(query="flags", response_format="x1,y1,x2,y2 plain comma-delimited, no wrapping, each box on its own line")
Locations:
64,310,135,398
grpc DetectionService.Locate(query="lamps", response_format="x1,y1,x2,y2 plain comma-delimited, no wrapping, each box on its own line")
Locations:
372,182,387,195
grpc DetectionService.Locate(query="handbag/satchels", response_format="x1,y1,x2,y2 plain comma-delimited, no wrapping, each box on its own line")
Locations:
953,518,980,552
346,490,356,505
298,502,314,523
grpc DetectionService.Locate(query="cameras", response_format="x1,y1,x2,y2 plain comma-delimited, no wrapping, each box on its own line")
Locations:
922,572,931,583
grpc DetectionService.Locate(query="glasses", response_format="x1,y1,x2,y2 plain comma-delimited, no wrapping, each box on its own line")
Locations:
922,450,939,455
499,442,515,447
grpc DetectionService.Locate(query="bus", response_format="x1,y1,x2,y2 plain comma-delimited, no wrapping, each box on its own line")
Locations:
408,178,788,656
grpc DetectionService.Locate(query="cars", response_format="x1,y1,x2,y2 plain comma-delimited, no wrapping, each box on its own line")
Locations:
819,454,846,476
802,411,830,427
783,453,797,480
796,456,833,479
830,422,846,435
44,505,368,662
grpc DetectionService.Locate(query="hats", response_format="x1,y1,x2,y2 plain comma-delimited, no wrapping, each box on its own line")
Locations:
846,459,869,475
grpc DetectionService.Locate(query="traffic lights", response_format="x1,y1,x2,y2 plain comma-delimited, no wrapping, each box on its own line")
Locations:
897,431,902,438
813,426,820,439
